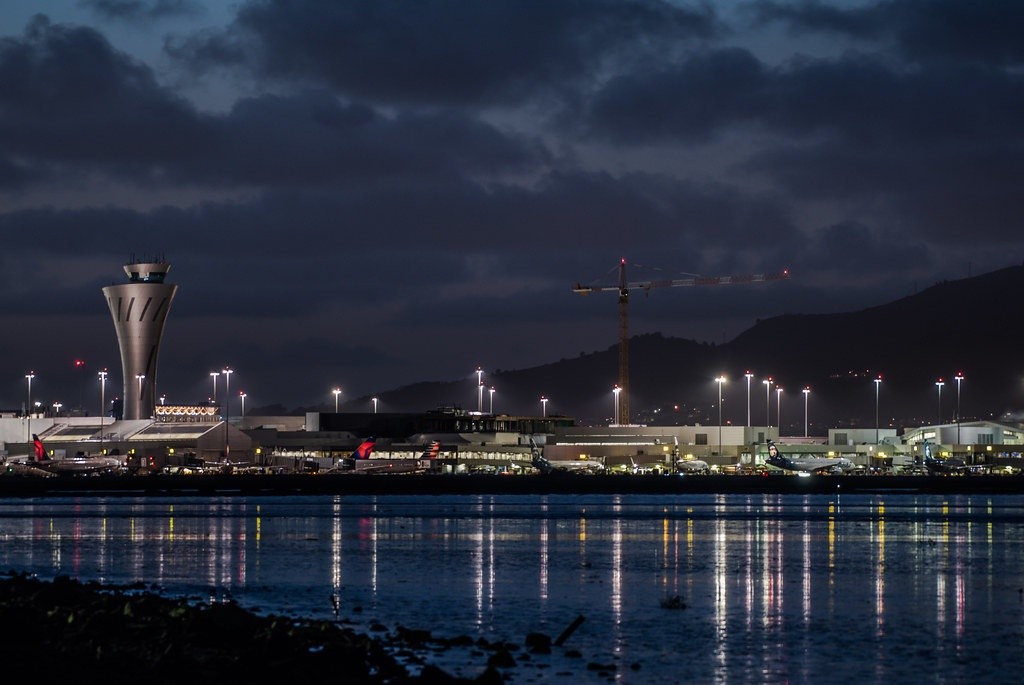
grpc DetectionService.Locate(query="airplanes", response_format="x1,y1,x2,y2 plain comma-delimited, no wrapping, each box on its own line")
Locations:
0,432,121,479
766,439,856,473
337,436,444,475
924,440,969,474
673,436,710,474
528,438,607,475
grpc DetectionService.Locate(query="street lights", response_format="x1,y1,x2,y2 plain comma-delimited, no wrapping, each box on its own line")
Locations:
714,378,726,455
613,388,623,425
762,379,774,425
802,389,810,438
873,379,882,446
239,393,247,420
210,372,222,403
52,402,63,418
372,397,379,414
333,390,341,413
541,399,548,416
936,382,945,425
25,374,36,445
955,376,966,445
97,370,107,456
775,388,783,427
475,370,484,413
744,373,756,427
488,388,496,413
221,369,234,466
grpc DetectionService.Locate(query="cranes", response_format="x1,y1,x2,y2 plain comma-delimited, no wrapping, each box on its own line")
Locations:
571,257,796,423
